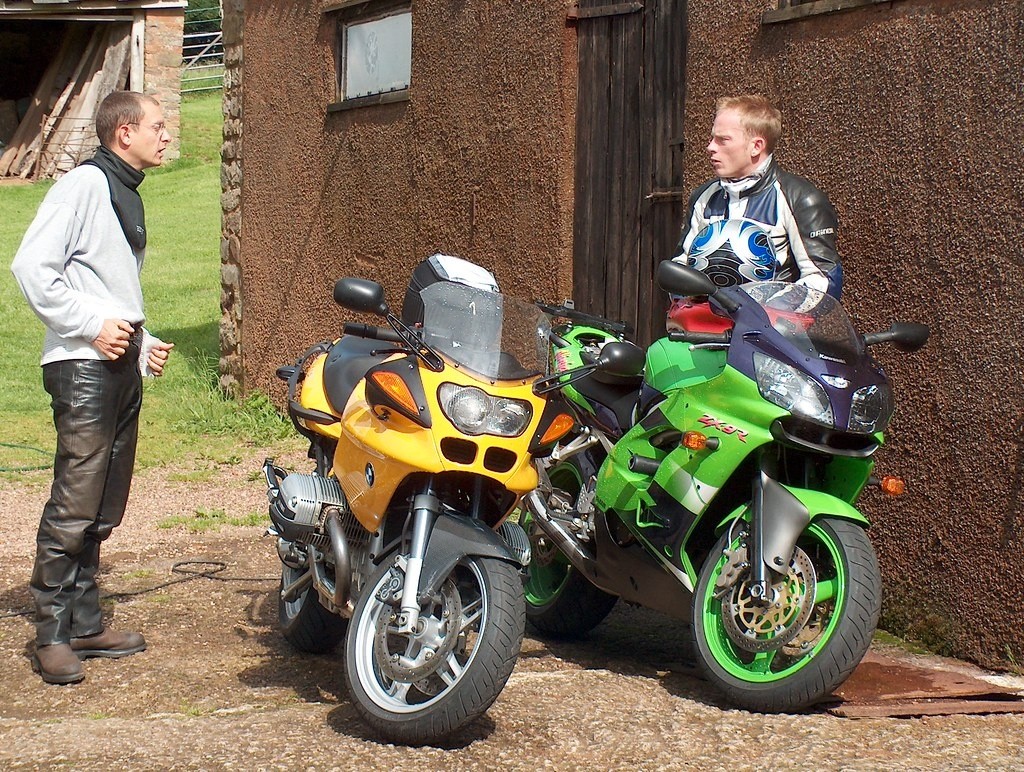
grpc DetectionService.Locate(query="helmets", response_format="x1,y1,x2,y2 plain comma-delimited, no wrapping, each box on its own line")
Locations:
686,220,776,304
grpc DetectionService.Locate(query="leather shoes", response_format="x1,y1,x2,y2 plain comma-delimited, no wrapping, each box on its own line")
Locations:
68,627,146,660
33,644,85,683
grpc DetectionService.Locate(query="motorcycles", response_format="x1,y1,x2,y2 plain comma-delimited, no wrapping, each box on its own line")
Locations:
515,258,930,714
256,274,645,747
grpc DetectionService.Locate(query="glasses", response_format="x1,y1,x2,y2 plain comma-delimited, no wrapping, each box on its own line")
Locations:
126,122,166,132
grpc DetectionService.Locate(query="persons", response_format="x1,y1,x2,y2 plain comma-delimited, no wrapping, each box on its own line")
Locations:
666,97,841,335
10,90,175,684
